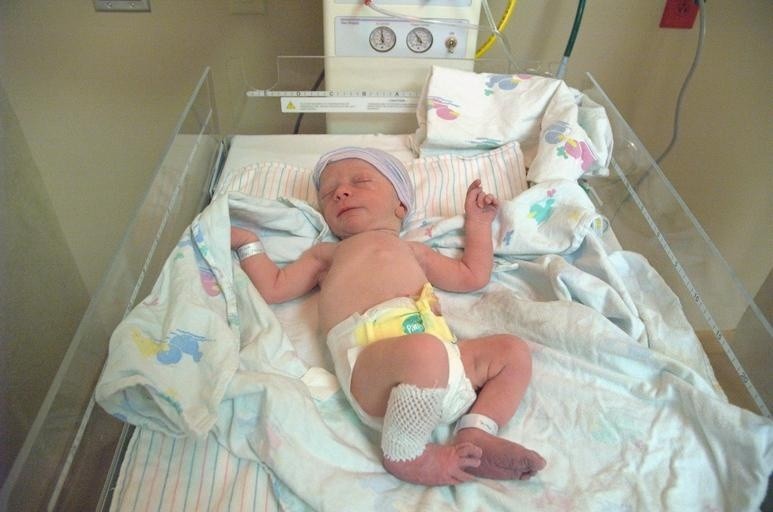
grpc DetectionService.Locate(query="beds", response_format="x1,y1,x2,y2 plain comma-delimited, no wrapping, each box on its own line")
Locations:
0,53,773,512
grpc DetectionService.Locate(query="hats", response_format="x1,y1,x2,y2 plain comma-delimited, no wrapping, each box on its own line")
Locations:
312,146,413,229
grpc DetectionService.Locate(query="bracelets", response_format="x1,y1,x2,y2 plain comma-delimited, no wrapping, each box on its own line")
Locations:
233,239,266,264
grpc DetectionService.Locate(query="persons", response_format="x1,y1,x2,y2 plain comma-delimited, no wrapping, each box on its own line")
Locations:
227,144,550,488
